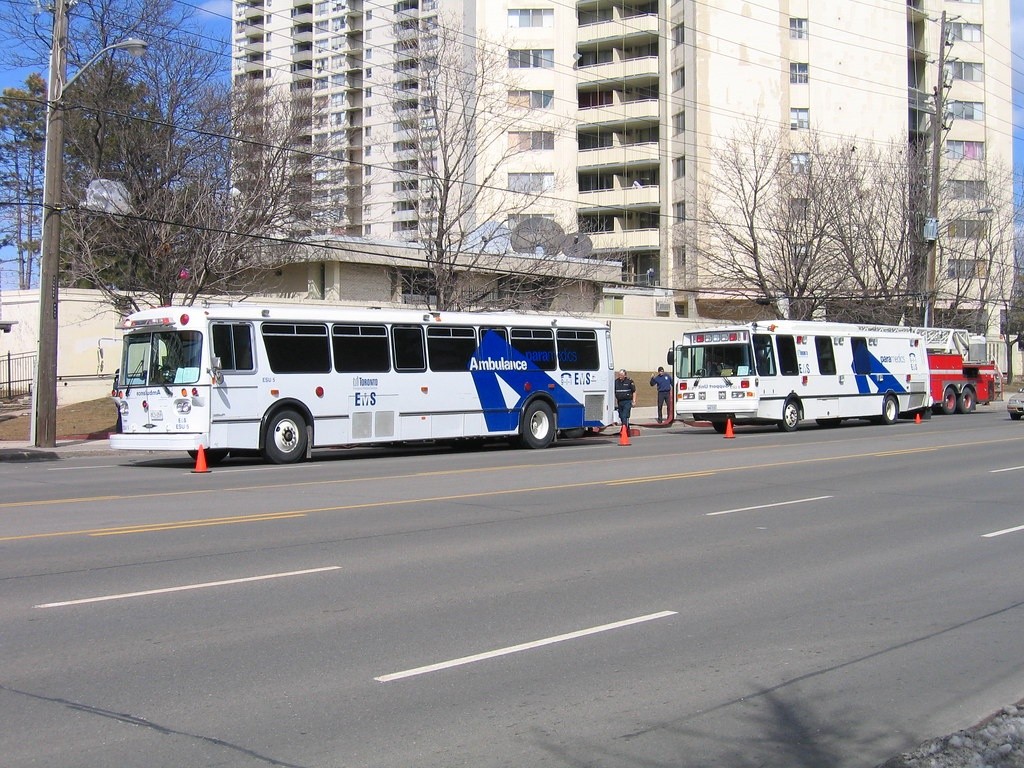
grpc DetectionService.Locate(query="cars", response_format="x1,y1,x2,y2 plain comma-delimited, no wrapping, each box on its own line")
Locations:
1007,387,1024,420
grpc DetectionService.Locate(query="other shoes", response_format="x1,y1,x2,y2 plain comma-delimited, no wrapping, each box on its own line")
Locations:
627,431,630,436
658,418,662,423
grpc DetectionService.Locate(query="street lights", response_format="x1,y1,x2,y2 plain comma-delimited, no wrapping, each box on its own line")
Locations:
32,37,147,448
924,207,992,327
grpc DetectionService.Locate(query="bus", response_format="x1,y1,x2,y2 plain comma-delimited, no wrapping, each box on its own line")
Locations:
666,320,934,434
97,304,615,463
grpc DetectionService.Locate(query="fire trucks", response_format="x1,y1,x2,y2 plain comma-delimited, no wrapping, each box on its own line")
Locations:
857,324,1005,419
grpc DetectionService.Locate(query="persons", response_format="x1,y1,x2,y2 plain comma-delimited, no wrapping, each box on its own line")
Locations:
615,369,636,427
650,367,673,423
113,369,123,434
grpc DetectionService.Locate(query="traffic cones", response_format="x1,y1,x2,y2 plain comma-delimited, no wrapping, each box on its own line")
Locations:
617,425,632,446
723,419,736,438
191,445,211,473
914,413,922,424
